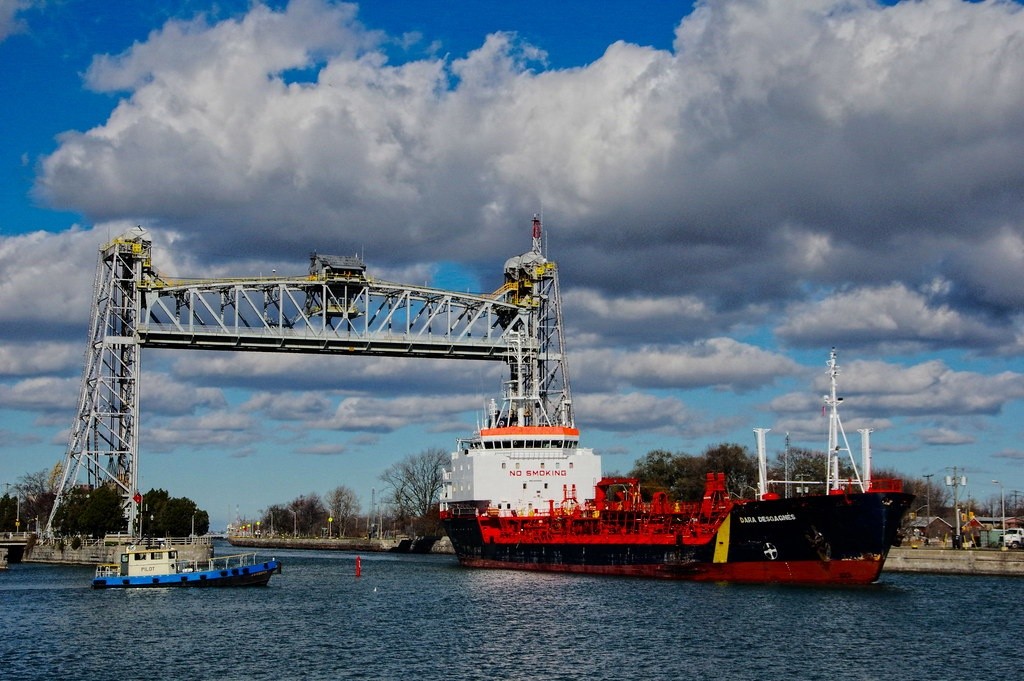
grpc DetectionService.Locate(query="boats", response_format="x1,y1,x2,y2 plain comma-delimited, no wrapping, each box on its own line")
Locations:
91,502,281,590
439,326,903,586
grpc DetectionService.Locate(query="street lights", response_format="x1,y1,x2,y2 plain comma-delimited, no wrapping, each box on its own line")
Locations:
5,483,20,534
991,480,1005,547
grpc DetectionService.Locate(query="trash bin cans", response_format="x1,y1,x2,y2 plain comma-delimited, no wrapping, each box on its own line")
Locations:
951,534,961,549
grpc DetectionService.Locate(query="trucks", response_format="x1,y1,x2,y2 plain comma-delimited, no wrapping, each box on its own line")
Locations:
1006,527,1024,550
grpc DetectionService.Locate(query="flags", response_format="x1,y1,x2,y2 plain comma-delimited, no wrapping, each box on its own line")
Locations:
820,405,825,418
